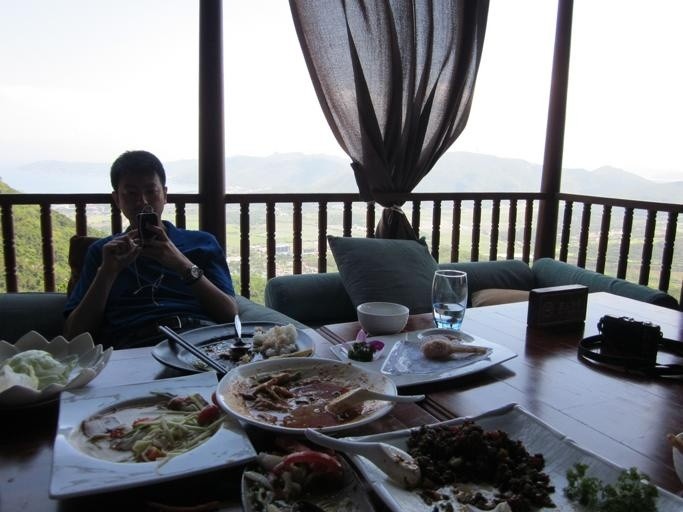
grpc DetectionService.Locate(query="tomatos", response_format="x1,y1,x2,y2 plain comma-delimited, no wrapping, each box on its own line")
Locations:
269,450,344,501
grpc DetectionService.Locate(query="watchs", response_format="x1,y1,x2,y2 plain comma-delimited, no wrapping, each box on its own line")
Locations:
179,264,204,286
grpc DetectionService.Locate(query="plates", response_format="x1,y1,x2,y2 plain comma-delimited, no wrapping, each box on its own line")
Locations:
51,370,256,502
216,356,399,432
330,329,518,387
241,449,376,512
0,331,114,403
339,402,682,511
151,322,318,371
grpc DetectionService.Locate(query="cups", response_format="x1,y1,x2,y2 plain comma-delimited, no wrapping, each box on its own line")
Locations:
430,268,468,332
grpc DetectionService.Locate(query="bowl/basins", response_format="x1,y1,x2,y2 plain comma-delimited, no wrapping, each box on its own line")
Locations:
357,302,410,335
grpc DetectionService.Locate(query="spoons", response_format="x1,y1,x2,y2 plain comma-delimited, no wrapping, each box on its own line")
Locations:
329,388,429,411
305,424,423,484
231,313,252,352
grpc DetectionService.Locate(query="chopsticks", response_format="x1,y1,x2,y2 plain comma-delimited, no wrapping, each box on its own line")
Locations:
158,324,228,374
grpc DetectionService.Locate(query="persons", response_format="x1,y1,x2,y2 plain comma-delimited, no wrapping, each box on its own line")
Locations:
62,150,239,351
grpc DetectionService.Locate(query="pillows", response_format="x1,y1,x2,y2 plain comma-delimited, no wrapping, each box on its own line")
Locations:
326,235,459,320
470,288,532,307
65,235,107,309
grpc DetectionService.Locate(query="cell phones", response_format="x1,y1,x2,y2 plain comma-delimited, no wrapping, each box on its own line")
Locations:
137,213,158,250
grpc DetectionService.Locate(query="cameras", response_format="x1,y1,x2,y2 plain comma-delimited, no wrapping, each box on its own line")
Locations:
597,314,663,364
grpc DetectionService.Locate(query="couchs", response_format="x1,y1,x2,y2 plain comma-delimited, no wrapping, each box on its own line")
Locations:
265,257,681,330
0,288,313,354
